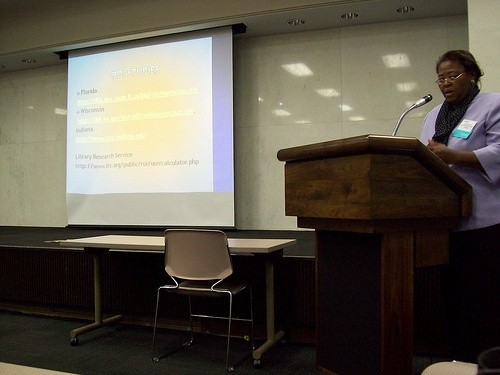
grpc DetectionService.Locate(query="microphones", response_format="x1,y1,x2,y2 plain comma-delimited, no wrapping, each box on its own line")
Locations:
392,94,433,136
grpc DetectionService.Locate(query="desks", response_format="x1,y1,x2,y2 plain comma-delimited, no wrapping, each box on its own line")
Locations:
58,234,296,366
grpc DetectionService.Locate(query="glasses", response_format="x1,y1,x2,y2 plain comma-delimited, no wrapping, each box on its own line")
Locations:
435,70,469,84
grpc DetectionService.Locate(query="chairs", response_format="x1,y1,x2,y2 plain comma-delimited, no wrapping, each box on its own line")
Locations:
150,228,253,371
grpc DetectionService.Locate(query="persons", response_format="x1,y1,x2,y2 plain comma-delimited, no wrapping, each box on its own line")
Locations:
417,49,500,364
